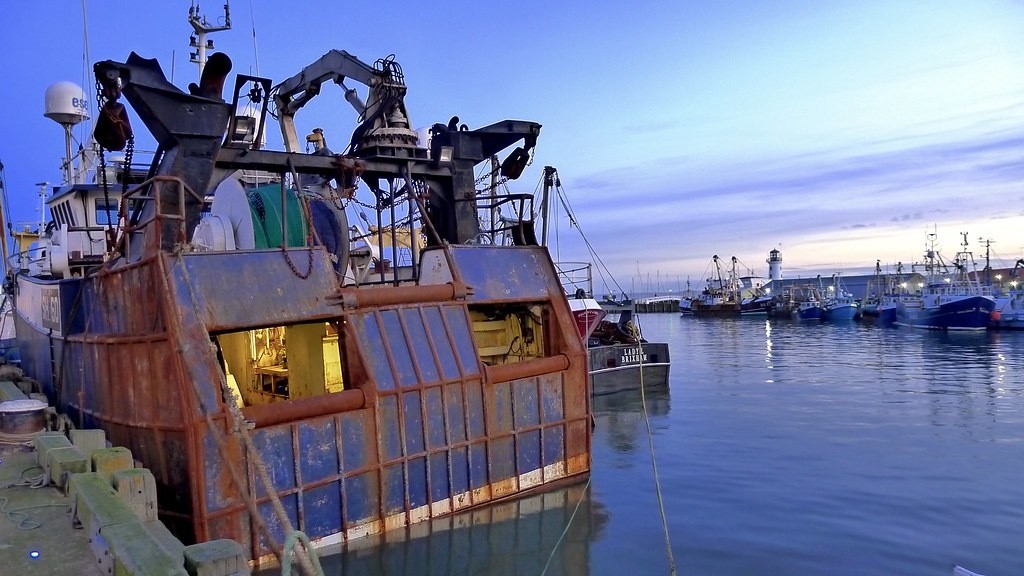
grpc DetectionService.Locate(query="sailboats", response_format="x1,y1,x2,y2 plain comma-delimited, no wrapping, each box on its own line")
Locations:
511,164,672,395
602,231,1024,330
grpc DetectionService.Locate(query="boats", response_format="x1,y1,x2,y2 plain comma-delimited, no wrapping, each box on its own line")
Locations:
0,0,595,575
